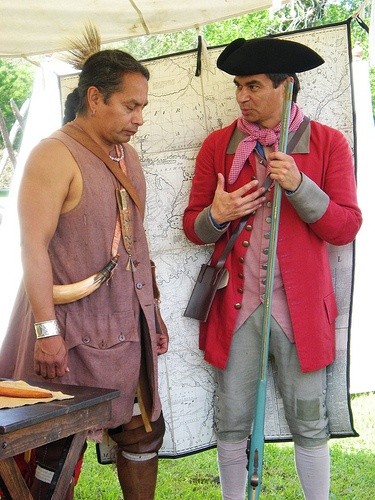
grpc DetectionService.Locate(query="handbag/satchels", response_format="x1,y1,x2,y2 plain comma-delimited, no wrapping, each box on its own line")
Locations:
180,263,232,322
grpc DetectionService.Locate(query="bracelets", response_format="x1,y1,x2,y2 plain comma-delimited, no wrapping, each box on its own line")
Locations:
34,320,61,339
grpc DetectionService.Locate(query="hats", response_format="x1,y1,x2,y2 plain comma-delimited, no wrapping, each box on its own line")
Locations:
216,38,327,76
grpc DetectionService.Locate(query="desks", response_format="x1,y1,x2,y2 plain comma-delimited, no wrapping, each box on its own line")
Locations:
0,375,121,500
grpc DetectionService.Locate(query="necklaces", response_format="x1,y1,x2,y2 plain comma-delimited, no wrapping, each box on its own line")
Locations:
107,144,125,161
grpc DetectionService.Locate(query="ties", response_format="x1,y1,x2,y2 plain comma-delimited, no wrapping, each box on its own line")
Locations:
224,103,304,186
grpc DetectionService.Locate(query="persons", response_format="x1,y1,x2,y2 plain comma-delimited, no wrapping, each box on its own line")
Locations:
0,49,169,500
182,38,363,500
